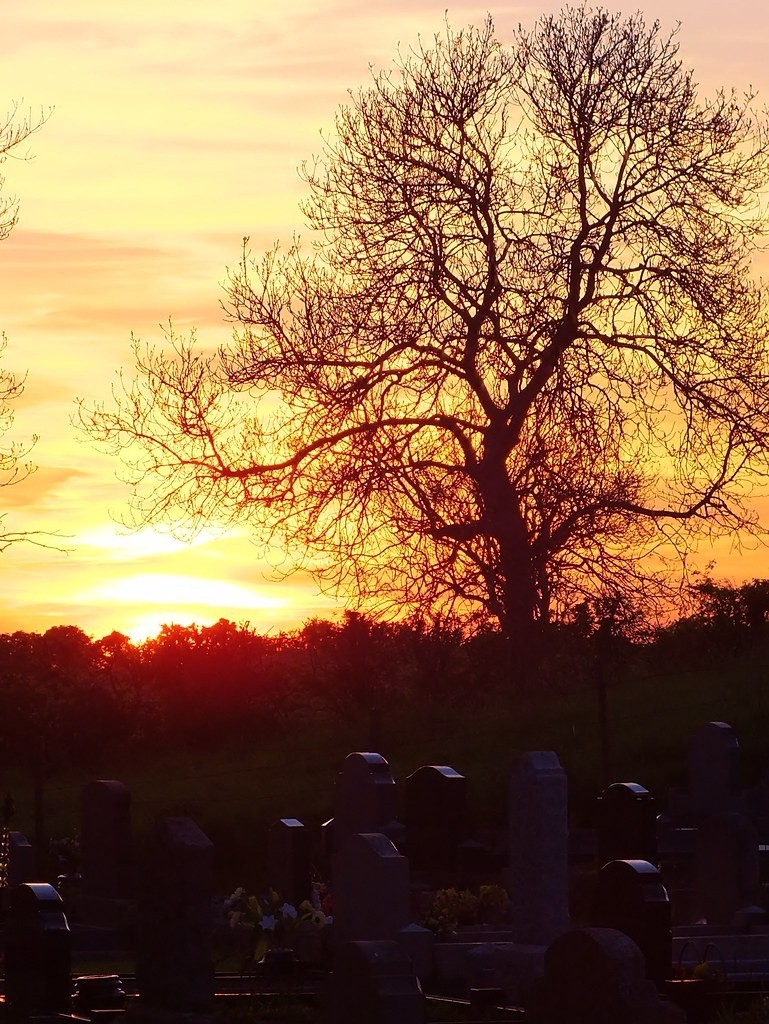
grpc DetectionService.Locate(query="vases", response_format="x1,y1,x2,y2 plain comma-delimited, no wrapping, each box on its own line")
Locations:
458,909,481,926
259,941,302,977
77,974,126,1016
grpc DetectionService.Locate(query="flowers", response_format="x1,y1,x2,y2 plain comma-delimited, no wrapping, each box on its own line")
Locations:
671,956,736,993
217,873,349,938
417,878,511,940
